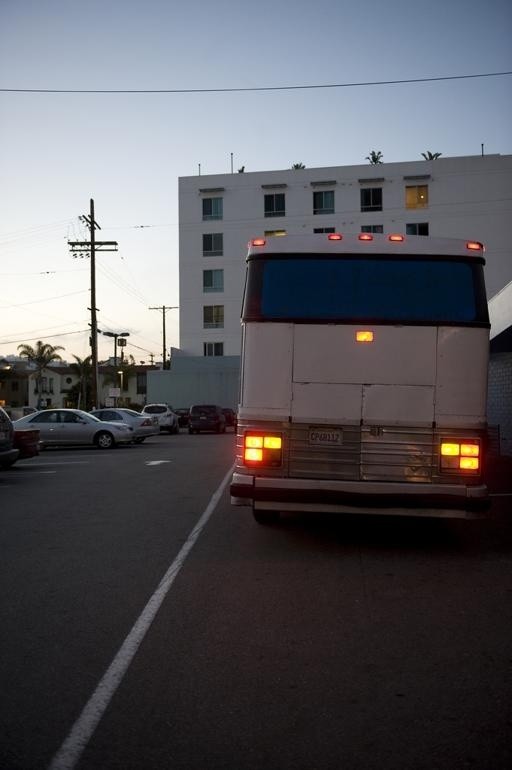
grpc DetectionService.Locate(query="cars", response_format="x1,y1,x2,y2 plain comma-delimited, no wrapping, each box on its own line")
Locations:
0,402,236,472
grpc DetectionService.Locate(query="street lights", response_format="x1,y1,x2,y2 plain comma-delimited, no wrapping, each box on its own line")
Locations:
118,370,124,400
101,330,131,407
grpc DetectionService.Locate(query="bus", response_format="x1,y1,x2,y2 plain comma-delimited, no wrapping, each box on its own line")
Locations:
222,230,499,535
